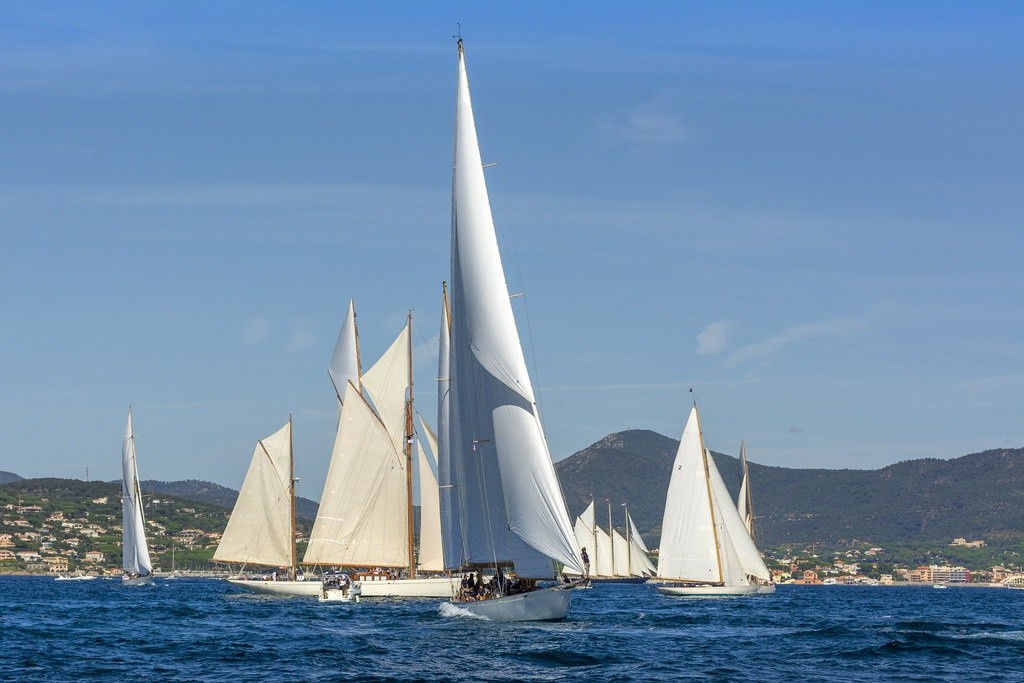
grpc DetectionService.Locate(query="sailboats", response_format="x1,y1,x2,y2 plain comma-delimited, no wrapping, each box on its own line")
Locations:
209,22,776,626
121,402,157,588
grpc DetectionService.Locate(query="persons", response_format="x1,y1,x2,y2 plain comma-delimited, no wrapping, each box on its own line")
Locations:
563,575,571,589
582,546,590,573
461,566,523,602
241,568,406,580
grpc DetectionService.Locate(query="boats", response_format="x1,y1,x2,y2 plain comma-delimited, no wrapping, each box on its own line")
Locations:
54,572,98,582
